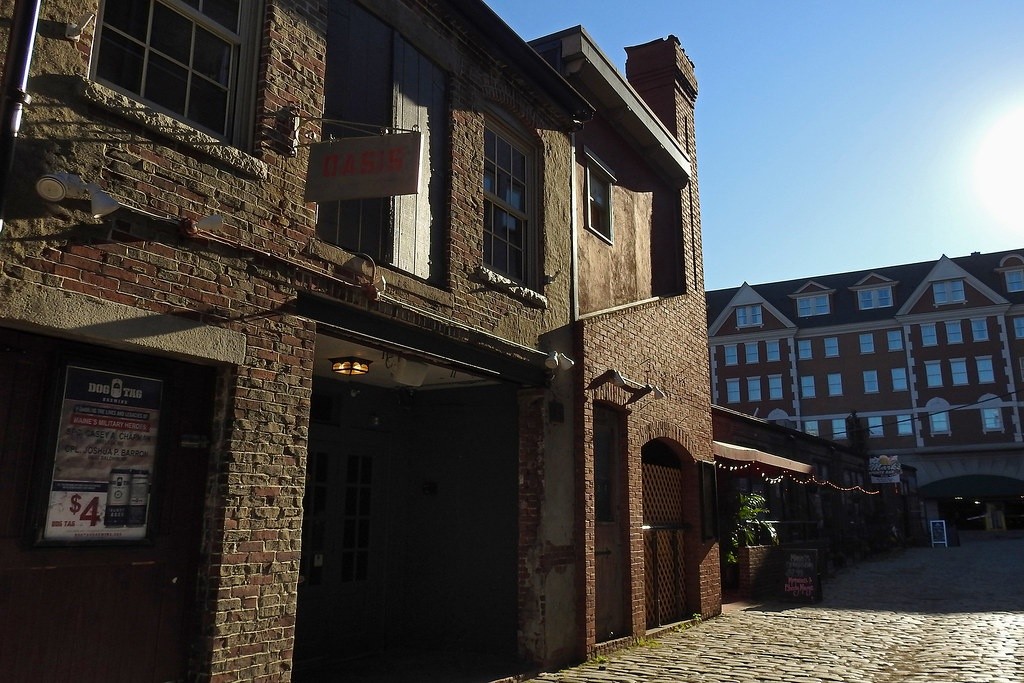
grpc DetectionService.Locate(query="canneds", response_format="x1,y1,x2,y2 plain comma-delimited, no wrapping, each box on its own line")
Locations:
104,467,150,528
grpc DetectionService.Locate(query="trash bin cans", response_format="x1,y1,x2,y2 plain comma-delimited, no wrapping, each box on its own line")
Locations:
784,548,824,604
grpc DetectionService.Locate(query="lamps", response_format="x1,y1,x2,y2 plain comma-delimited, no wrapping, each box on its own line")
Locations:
36,174,120,218
180,215,223,237
646,385,665,399
611,369,626,386
327,356,374,376
545,351,558,369
557,353,574,371
359,253,387,301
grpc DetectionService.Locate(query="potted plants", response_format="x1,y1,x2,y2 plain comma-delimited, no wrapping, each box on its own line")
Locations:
726,488,782,598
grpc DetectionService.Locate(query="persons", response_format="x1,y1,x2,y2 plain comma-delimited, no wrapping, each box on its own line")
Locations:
799,484,824,538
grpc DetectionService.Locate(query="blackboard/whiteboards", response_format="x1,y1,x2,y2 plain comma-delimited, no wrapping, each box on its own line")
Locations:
930,520,947,543
